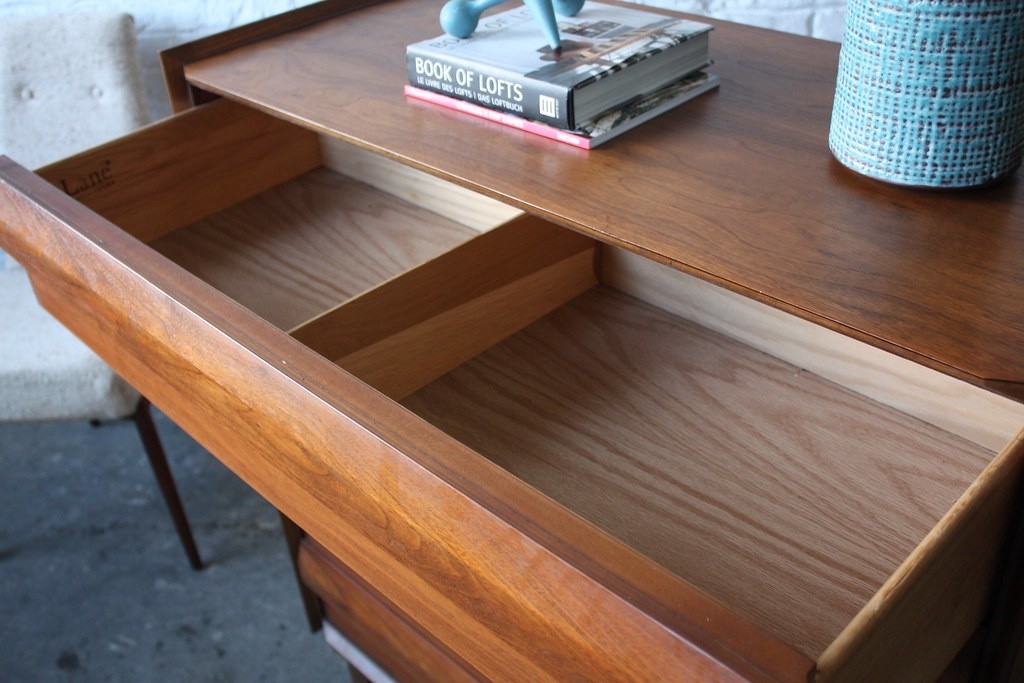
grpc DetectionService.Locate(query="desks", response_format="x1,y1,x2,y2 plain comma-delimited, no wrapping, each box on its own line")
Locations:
0,0,1024,683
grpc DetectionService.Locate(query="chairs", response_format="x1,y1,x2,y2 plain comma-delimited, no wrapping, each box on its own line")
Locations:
0,9,203,572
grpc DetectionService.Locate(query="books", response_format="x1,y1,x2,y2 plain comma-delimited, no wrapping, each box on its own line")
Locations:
404,72,722,150
406,0,714,130
